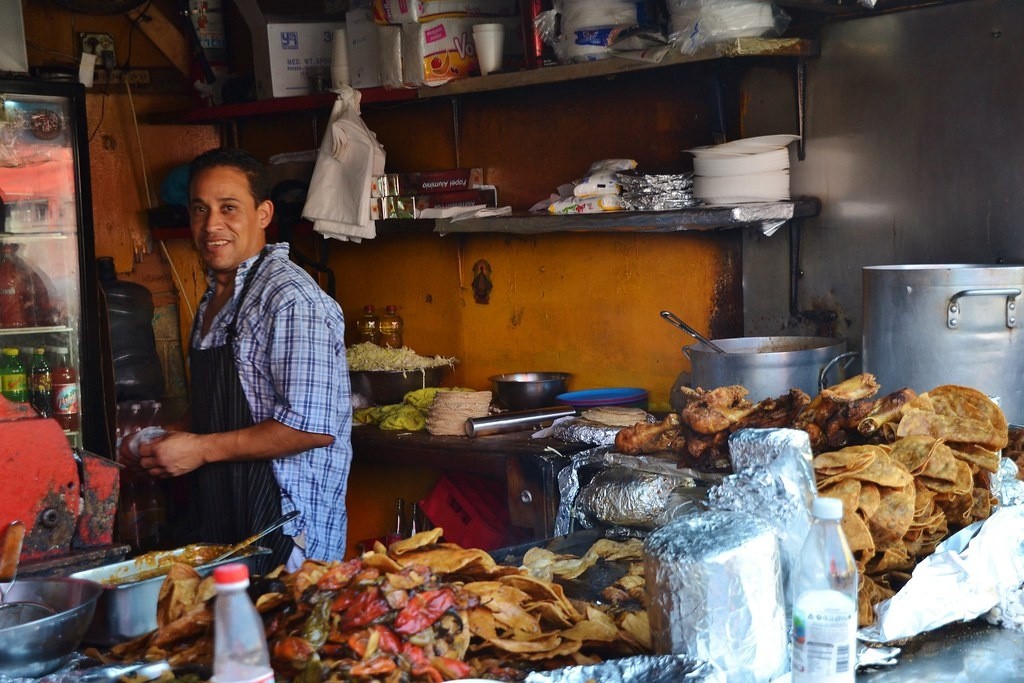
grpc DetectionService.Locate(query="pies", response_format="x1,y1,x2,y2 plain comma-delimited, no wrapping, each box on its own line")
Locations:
581,406,646,427
811,384,1010,626
425,390,493,436
362,526,652,667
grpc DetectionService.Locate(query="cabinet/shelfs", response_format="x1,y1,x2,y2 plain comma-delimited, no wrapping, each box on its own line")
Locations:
152,37,829,251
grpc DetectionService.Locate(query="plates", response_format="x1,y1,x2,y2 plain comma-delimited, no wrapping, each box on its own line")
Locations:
680,134,801,205
666,0,775,37
560,0,639,62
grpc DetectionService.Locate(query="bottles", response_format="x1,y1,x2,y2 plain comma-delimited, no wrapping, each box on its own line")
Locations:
0,346,80,432
97,256,164,401
357,305,379,344
393,499,404,535
422,515,431,531
379,305,403,348
213,564,274,683
407,503,420,538
793,497,858,683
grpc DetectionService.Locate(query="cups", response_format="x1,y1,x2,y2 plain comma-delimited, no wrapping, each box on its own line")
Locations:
331,29,350,90
473,23,504,76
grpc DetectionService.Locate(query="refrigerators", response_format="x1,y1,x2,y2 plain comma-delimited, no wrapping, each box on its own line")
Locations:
0,77,104,456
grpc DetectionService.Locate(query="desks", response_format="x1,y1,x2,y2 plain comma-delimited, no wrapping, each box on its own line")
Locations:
350,402,671,544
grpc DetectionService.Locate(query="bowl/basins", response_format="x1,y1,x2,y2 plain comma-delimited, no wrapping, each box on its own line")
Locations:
488,372,573,411
312,74,331,91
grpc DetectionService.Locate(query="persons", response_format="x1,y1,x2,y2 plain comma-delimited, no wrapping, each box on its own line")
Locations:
119,146,353,578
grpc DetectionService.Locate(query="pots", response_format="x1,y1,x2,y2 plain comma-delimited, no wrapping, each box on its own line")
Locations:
863,264,1024,425
682,336,859,403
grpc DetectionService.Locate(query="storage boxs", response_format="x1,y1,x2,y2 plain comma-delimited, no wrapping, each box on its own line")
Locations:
239,1,349,102
